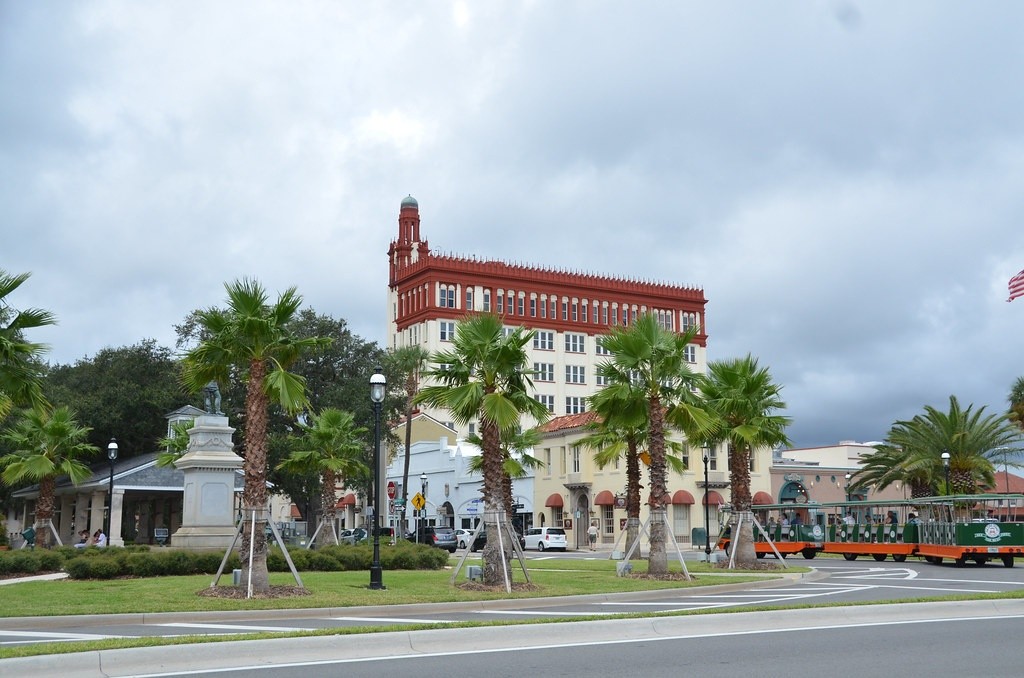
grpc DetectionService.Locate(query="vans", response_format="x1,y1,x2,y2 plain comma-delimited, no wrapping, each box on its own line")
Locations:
525,527,567,552
372,527,409,542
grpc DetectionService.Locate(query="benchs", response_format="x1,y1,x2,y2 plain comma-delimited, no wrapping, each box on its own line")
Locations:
759,524,904,543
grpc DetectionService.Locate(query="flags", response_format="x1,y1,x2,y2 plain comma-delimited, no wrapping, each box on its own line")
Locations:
1007,269,1024,302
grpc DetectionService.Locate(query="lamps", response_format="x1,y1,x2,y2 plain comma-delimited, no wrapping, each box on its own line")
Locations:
589,494,596,517
563,495,569,517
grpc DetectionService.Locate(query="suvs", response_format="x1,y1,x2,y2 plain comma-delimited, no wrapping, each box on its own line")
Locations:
406,526,458,553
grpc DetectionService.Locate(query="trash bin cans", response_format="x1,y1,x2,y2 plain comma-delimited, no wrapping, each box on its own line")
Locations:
692,527,707,550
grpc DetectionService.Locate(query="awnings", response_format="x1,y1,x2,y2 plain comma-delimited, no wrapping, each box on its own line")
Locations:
702,491,724,504
594,490,614,505
648,493,671,504
672,490,695,504
753,492,774,504
545,493,563,507
342,494,355,505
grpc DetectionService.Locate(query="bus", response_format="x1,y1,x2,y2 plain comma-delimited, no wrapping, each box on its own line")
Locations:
717,493,1024,567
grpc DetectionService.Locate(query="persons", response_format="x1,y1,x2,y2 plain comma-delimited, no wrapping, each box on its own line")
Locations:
865,515,875,524
791,513,803,524
781,513,789,525
74,529,106,548
882,511,898,524
909,513,924,523
587,523,599,551
837,512,855,524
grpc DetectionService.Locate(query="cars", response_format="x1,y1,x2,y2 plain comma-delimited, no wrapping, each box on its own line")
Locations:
339,529,367,543
471,529,525,551
453,529,478,549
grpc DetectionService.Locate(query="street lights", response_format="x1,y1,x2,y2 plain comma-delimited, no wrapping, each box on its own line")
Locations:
105,438,119,546
941,449,951,495
421,473,427,543
701,442,713,562
845,471,852,501
370,363,387,589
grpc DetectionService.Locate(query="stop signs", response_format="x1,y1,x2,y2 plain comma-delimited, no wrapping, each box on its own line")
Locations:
387,482,395,499
